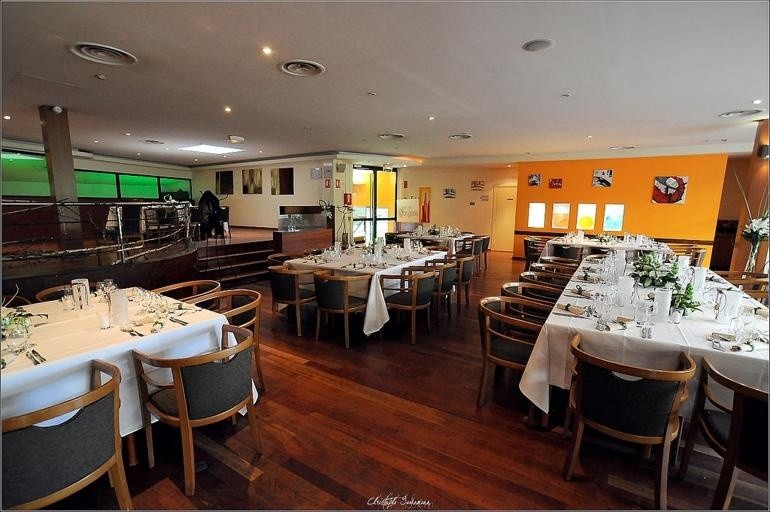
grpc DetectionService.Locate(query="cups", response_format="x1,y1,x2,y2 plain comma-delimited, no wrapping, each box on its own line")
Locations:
5,323,27,354
303,237,423,270
61,279,168,329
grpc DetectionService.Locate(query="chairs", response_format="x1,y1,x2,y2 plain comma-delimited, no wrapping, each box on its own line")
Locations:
152,279,221,311
2,295,31,308
132,324,263,496
0,359,134,510
680,357,768,510
184,288,265,392
35,281,97,302
267,224,492,348
92,205,231,266
478,231,770,467
562,333,696,510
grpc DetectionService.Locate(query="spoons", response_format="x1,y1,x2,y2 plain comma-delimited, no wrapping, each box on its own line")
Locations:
119,317,188,337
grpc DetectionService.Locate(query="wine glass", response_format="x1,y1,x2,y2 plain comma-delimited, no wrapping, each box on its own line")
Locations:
557,229,654,248
594,249,754,347
412,225,461,238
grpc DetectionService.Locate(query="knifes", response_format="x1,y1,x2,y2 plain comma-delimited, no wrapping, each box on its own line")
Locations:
25,351,40,366
32,350,47,361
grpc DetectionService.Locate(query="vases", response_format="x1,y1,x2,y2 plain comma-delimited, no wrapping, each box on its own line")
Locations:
744,242,759,273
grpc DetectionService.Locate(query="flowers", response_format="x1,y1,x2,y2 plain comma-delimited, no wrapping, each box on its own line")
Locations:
741,210,769,271
